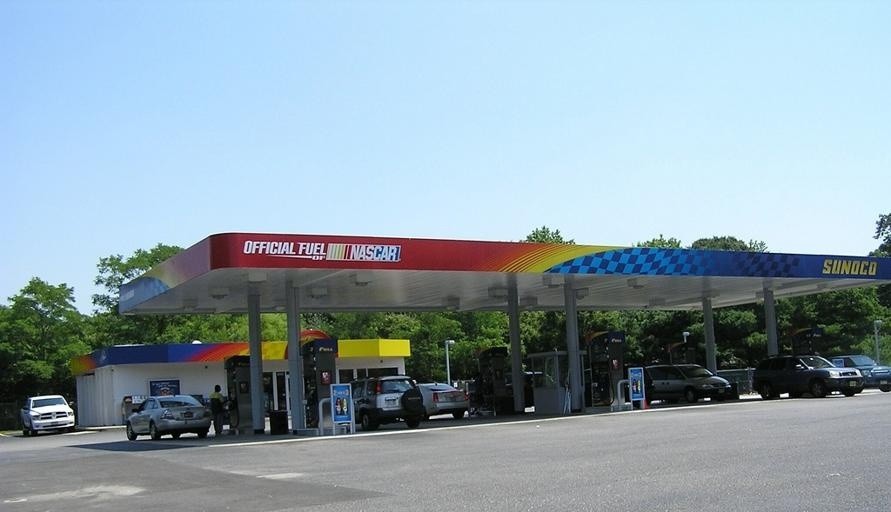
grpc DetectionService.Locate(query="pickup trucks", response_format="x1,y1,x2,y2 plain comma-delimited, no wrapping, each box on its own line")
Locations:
20,395,76,436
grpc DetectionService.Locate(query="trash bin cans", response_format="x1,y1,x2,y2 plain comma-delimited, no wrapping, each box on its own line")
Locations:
269,410,288,435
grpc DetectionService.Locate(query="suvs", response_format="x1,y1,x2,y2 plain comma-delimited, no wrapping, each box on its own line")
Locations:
352,374,423,430
752,354,865,398
829,354,890,392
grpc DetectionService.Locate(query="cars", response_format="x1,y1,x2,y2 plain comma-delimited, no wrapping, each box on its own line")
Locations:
416,382,469,420
126,394,211,440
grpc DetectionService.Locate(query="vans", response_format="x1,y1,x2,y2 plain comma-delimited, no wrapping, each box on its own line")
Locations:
644,364,730,403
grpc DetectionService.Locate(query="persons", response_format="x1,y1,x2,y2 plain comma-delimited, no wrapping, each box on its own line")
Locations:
210,384,227,438
